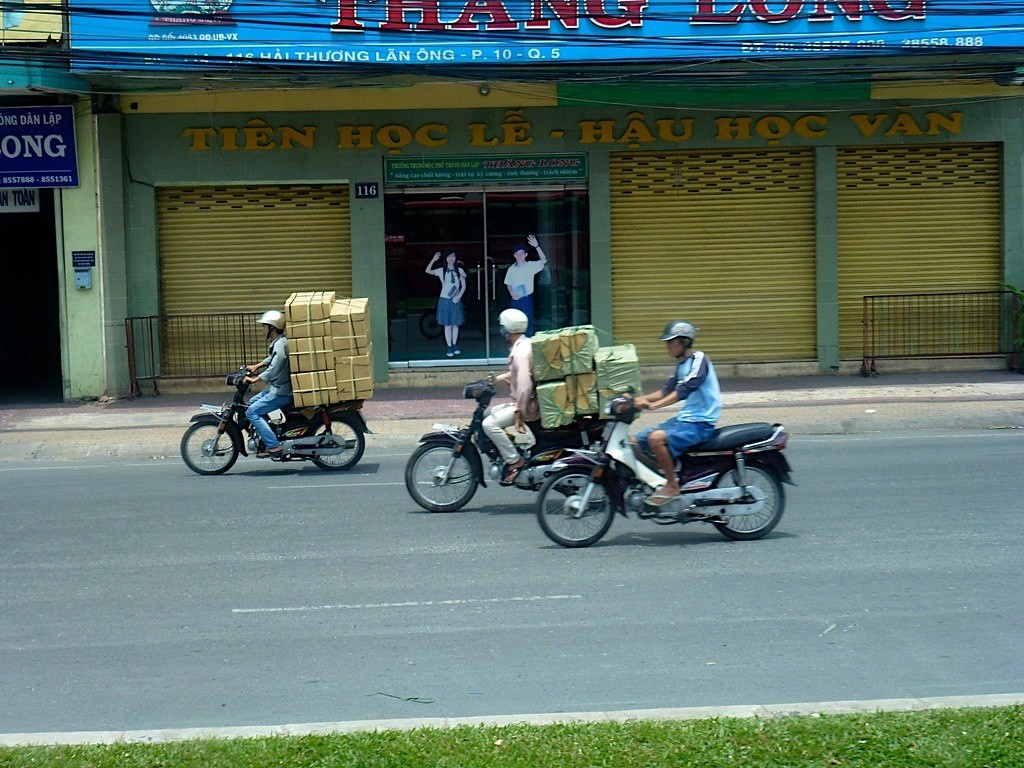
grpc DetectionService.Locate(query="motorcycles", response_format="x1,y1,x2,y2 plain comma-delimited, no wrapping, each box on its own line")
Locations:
534,386,800,548
178,364,378,475
419,282,513,338
403,371,628,513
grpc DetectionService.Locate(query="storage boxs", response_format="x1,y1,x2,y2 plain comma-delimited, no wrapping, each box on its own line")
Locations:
284,292,374,407
531,323,642,428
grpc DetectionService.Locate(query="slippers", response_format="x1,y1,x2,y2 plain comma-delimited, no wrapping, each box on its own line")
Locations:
643,491,681,506
500,462,527,486
256,450,284,458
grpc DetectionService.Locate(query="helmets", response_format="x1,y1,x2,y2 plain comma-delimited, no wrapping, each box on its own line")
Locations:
499,309,528,333
257,311,285,329
659,320,695,340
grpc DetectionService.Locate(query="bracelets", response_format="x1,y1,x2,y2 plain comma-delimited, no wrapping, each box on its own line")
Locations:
535,245,539,248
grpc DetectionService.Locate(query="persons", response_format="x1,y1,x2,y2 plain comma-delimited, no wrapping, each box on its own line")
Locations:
503,234,547,337
623,320,722,507
482,309,541,486
425,251,467,357
242,310,291,458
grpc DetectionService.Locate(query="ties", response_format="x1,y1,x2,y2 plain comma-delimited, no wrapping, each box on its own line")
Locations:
449,270,454,283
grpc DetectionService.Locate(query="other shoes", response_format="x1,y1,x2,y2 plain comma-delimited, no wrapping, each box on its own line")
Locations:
447,347,454,357
453,345,460,355
